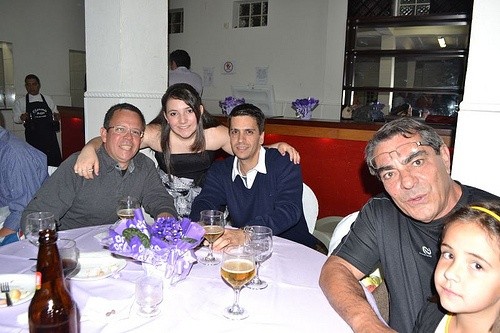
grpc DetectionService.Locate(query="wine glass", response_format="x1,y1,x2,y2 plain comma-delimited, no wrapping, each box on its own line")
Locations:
198,209,225,267
220,244,257,320
244,225,273,291
24,211,56,272
134,276,163,319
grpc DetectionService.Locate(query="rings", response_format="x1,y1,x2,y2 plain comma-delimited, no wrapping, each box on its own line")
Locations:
88,169,93,172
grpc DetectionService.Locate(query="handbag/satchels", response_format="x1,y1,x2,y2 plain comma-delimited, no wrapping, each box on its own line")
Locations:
351,101,385,122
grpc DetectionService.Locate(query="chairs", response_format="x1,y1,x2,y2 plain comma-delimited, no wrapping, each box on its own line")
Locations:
328,208,359,256
302,180,318,234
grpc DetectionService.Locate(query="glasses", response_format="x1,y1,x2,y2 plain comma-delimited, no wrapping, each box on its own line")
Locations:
369,141,429,170
107,126,143,137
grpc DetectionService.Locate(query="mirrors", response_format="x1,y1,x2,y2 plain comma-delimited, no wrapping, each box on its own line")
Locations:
0,39,17,110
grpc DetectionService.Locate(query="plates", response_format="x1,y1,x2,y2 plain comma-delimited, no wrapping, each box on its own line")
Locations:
0,273,36,308
66,252,127,281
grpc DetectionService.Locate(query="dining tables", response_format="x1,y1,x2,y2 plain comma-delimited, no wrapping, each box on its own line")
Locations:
0,217,389,333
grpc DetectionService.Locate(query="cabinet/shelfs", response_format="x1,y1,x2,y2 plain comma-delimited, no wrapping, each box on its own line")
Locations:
339,13,472,122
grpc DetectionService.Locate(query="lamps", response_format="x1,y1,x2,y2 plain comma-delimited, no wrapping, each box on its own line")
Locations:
436,36,448,48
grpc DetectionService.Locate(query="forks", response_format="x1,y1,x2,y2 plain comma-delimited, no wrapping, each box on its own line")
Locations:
1,281,13,306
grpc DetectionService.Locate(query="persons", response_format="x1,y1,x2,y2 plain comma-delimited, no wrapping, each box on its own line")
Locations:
0,125,52,245
19,104,178,239
190,103,328,256
319,117,500,333
411,200,500,333
167,49,204,100
74,82,301,219
12,73,63,167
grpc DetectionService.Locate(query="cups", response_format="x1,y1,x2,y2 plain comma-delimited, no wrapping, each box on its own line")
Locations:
57,237,77,279
116,197,141,221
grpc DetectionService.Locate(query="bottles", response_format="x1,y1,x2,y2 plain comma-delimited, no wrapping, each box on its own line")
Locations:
27,228,79,333
